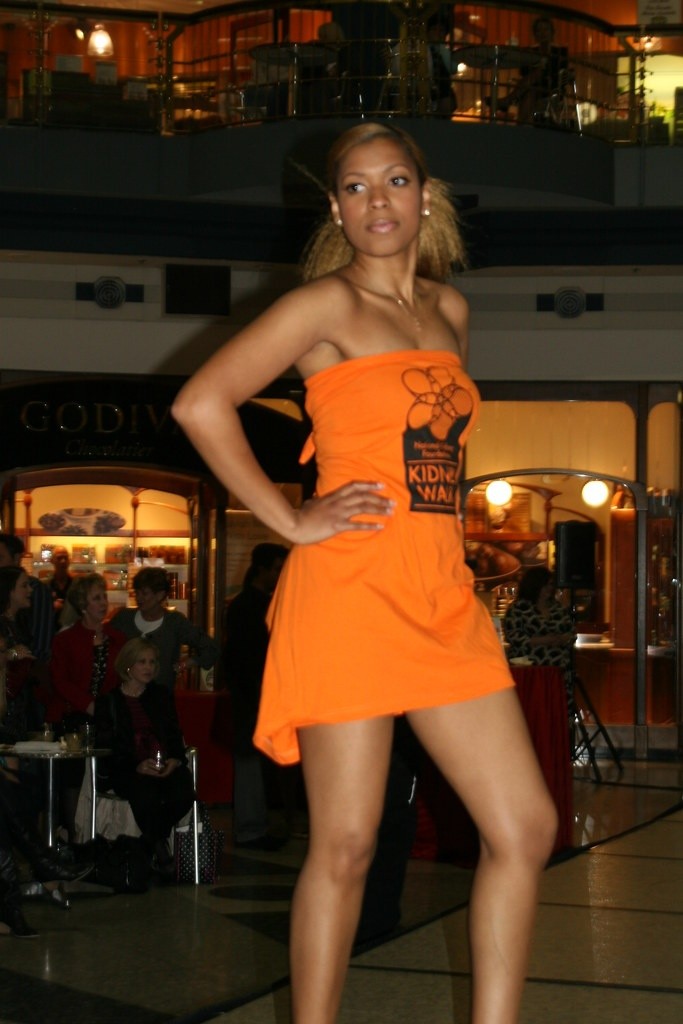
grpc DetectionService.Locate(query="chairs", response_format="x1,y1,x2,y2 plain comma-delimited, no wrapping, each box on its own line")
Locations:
537,69,586,133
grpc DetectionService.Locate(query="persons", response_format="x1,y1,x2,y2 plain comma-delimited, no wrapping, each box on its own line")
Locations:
486,17,569,122
171,125,559,1024
0,533,291,938
267,13,458,120
352,714,423,947
504,566,577,763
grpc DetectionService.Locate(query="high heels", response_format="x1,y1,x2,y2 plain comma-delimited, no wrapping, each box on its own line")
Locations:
0,900,41,938
32,847,94,891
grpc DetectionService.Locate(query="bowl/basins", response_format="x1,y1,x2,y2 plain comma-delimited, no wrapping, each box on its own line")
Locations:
576,633,601,642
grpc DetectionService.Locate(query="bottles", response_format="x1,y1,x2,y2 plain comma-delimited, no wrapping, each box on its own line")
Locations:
651,525,673,646
178,644,200,691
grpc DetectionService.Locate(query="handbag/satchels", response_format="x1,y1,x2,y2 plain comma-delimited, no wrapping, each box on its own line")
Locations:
0,615,33,698
175,800,225,885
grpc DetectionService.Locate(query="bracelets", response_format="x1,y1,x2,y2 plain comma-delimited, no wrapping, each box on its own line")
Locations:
7,648,18,661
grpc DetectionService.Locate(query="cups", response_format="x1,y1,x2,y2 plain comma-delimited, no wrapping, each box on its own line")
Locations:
60,733,84,752
79,722,96,749
147,745,167,771
39,731,55,742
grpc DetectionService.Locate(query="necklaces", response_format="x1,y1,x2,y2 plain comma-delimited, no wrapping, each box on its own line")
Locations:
122,683,145,696
352,261,424,332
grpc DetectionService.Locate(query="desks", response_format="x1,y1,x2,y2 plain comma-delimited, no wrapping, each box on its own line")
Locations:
0,746,112,906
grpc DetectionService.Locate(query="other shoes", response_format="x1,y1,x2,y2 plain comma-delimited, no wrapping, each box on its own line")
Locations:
113,846,152,894
237,830,288,852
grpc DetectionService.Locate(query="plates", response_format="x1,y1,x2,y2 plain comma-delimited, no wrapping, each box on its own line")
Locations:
574,643,614,648
520,560,546,569
474,564,522,585
